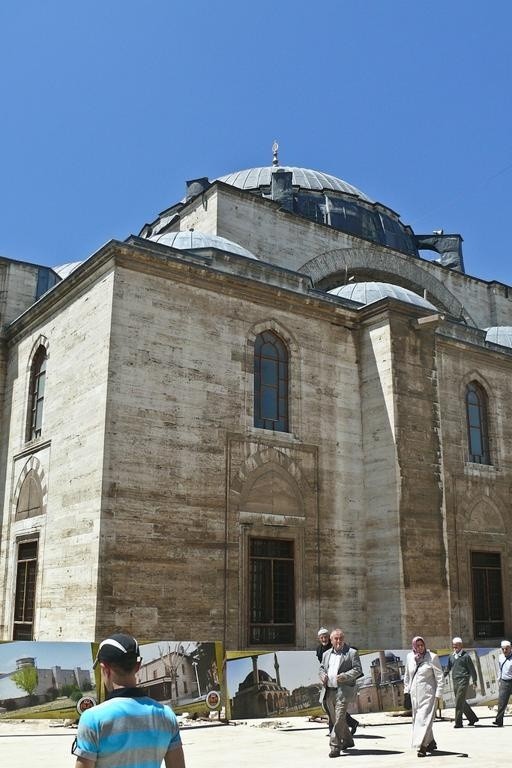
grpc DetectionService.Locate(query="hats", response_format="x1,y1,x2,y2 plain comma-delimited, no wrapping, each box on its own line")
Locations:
91,632,142,670
451,636,462,644
500,639,511,647
317,628,328,635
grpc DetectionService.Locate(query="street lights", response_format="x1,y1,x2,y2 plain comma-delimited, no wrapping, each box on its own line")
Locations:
192,662,202,697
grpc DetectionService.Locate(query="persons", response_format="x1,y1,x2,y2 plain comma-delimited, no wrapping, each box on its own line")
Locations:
404,636,444,757
73,632,189,768
493,639,512,728
444,637,479,728
315,626,364,758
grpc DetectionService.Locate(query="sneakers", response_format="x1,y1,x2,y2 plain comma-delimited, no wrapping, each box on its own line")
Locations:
325,720,359,758
453,717,502,728
417,741,437,757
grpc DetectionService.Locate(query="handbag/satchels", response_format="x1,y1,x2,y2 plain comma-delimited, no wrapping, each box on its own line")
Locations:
403,692,412,710
